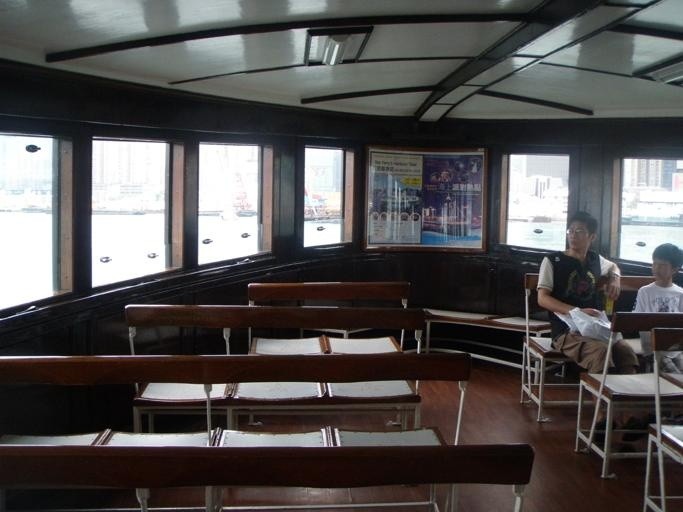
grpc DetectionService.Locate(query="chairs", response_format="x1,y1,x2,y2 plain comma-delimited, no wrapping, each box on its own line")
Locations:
1,272,534,512
490,265,683,512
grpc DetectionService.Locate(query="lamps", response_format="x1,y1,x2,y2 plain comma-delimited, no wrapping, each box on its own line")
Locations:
321,34,350,66
650,64,683,86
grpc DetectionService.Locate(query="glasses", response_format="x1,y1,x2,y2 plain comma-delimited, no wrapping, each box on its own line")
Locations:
566,228,585,234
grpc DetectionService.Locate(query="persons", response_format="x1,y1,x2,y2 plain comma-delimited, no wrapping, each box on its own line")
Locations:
537,211,657,443
632,243,683,425
